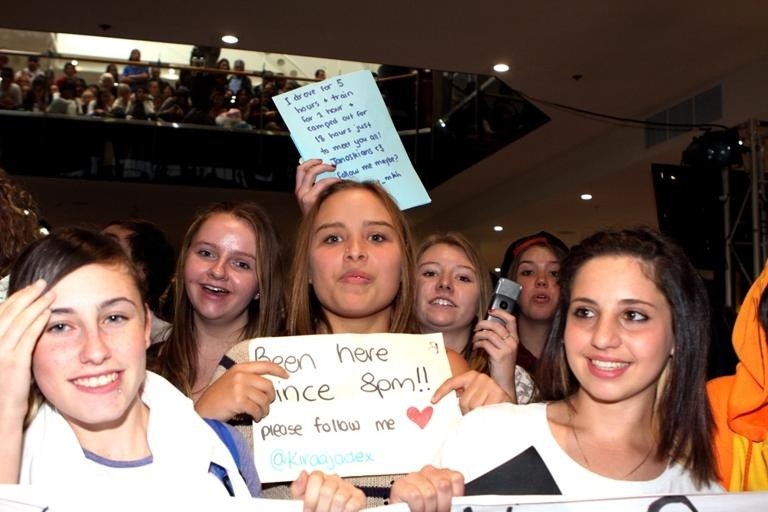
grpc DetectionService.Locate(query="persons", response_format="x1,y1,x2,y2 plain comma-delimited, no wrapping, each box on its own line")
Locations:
100,221,178,345
406,230,540,403
146,198,278,406
0,225,368,511
705,259,767,492
498,227,580,403
0,45,325,133
1,169,49,302
196,181,510,422
387,224,728,511
371,64,488,133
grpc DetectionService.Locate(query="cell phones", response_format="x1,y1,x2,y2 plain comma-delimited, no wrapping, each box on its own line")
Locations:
487,278,522,324
